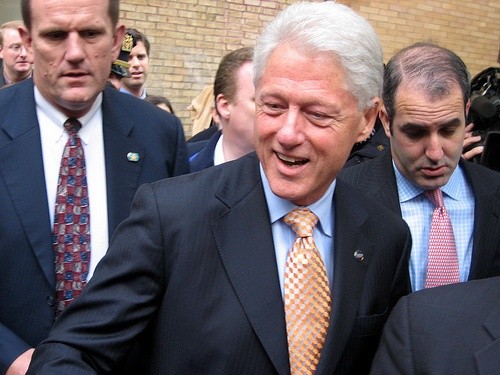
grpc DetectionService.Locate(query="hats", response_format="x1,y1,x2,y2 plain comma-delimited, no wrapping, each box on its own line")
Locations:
112,31,134,79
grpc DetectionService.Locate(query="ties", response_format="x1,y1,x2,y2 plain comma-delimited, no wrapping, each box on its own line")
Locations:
424,188,461,288
53,118,94,319
282,207,330,375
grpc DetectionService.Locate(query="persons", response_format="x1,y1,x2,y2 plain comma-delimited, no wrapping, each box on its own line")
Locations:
0,1,191,374
185,47,257,173
120,28,150,100
370,276,500,375
143,95,174,115
344,41,500,311
341,50,500,169
27,1,411,374
0,20,32,88
107,31,137,91
